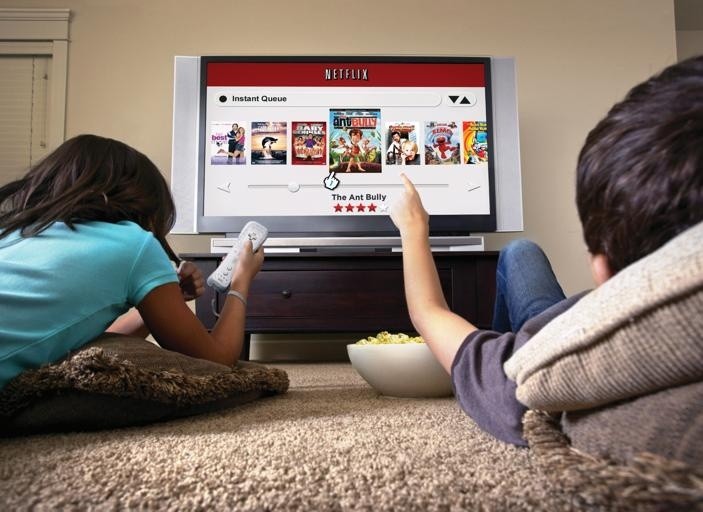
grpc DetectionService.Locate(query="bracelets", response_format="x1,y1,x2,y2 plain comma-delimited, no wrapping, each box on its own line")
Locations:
225,289,246,308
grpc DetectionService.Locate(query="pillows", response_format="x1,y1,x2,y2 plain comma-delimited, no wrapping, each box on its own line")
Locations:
0,328,290,437
520,381,703,504
504,221,702,409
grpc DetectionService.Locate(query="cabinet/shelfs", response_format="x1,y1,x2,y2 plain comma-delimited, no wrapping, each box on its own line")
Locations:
176,249,500,363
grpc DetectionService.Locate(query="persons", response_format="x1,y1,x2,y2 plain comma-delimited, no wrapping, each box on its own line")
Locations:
215,126,244,159
0,132,264,384
387,132,406,160
387,52,702,447
227,123,239,163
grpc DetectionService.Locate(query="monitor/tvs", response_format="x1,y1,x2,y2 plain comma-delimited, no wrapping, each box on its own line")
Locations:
170,54,526,253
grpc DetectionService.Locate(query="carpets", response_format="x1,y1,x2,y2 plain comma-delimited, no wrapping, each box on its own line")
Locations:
1,364,698,510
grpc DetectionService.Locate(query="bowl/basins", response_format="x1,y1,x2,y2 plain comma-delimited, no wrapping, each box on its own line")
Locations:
345,342,455,400
